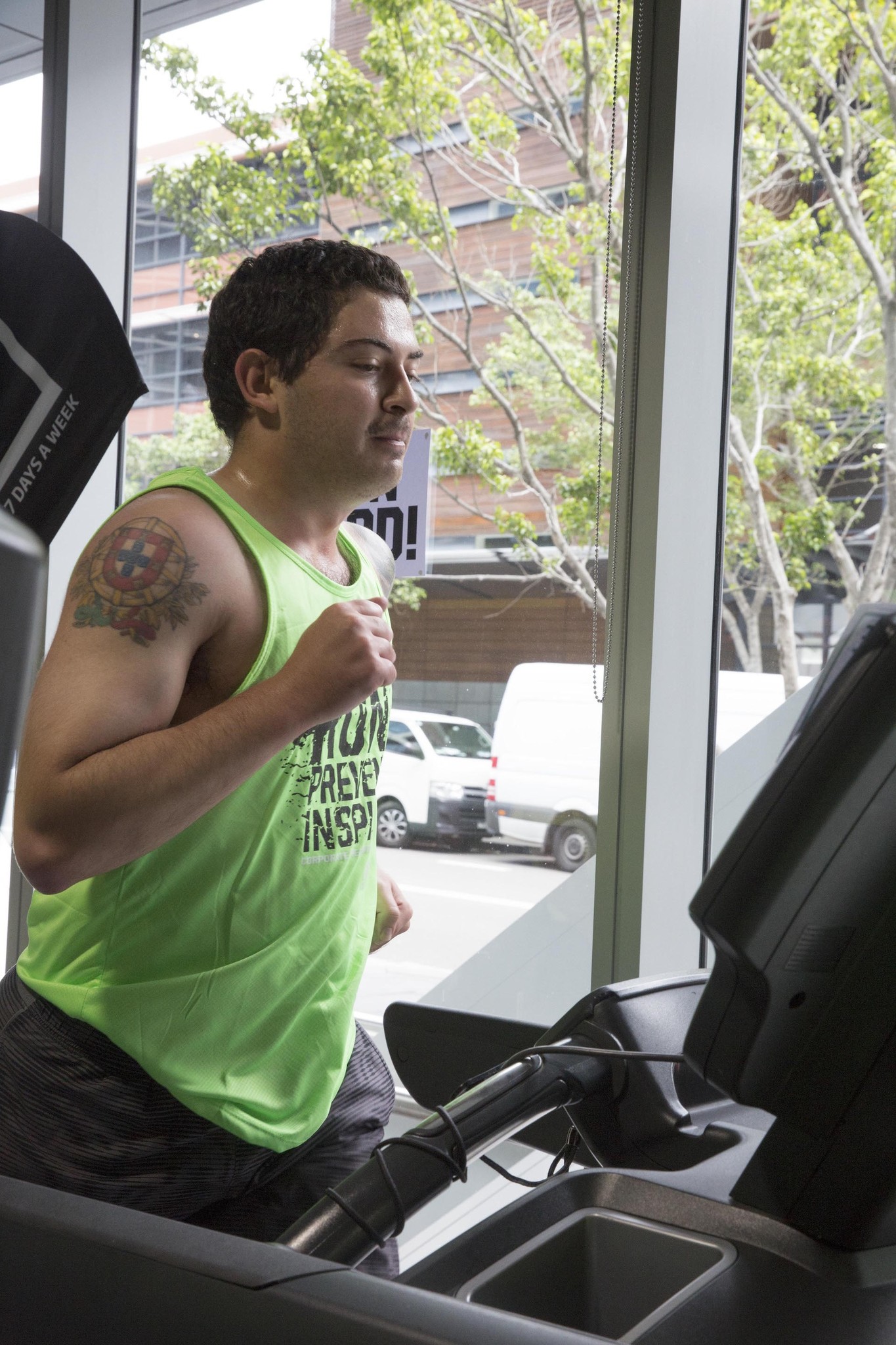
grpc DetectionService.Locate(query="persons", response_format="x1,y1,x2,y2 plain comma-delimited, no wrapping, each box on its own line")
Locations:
0,241,421,1276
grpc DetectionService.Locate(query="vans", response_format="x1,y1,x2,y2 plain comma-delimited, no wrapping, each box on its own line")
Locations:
481,662,809,874
376,709,492,853
